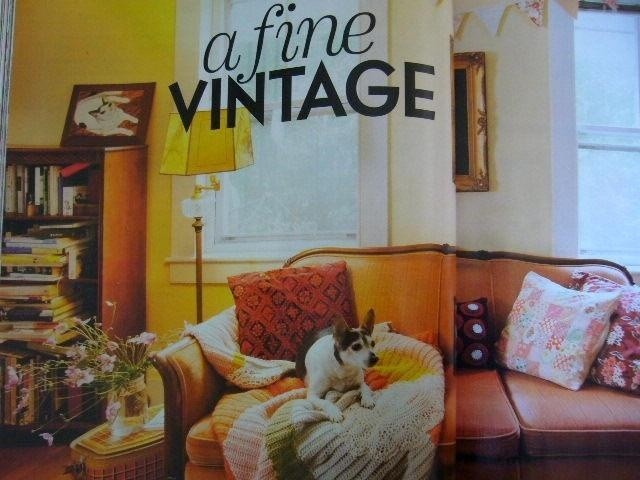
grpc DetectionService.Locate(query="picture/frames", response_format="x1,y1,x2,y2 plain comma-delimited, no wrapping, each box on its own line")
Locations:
57,83,155,148
450,52,489,191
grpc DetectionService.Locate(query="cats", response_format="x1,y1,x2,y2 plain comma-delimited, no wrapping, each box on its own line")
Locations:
74,89,138,140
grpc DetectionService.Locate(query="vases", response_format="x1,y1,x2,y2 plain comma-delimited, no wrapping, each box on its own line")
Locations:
107,373,149,434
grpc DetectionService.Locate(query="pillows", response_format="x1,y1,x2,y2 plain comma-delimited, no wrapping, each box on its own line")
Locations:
226,260,355,367
452,268,640,396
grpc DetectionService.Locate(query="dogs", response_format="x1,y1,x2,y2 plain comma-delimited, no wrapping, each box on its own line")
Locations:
293,306,380,425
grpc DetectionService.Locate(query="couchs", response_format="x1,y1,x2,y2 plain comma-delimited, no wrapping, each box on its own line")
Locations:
145,242,640,479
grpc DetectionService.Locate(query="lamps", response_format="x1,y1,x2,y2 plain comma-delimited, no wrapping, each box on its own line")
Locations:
157,107,255,325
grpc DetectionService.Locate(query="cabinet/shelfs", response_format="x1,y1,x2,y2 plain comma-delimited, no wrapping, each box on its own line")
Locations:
1,146,148,433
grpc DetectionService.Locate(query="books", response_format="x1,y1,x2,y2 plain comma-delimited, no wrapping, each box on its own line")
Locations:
0,164,97,425
143,408,164,431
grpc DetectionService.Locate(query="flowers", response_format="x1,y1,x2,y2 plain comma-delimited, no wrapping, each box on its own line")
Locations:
1,299,159,449
